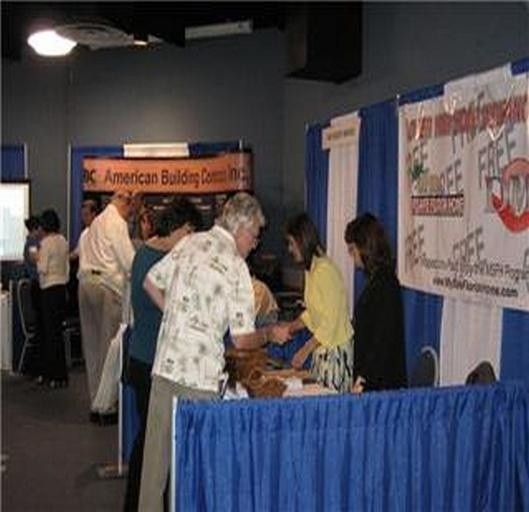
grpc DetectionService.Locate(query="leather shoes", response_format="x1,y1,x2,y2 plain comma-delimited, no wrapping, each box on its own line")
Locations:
90,407,118,427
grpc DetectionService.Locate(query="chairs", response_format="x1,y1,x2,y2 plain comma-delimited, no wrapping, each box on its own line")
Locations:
14,277,40,378
409,344,440,387
